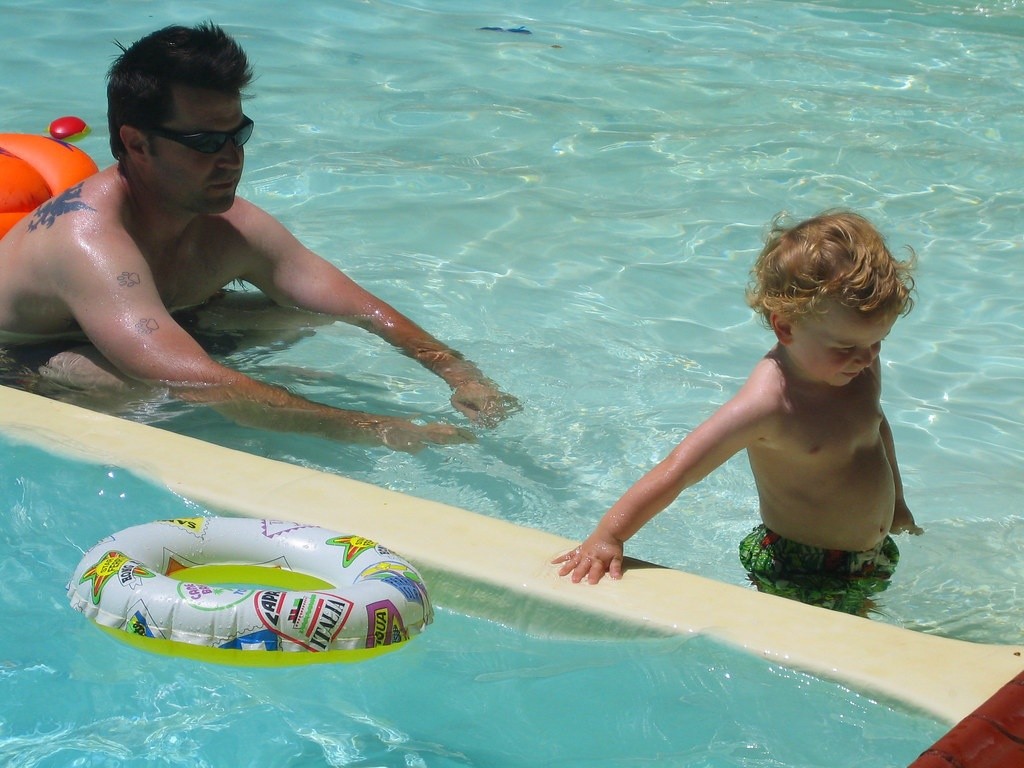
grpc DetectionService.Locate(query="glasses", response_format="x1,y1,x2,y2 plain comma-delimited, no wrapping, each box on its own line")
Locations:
141,106,254,154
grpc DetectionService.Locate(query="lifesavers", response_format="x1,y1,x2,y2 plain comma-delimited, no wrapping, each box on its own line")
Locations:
62,512,438,673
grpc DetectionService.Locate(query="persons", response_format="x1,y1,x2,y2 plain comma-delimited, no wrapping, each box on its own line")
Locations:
0,19,523,453
551,207,923,616
